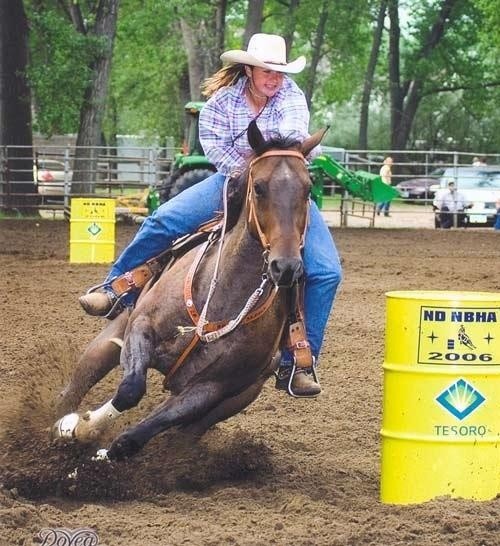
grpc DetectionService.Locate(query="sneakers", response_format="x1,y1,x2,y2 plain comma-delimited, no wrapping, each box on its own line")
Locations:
73,290,113,317
275,368,322,396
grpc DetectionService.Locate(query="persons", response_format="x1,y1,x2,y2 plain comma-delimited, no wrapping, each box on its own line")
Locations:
376,156,393,216
440,181,468,228
76,31,342,393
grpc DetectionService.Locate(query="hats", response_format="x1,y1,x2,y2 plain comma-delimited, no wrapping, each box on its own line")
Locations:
220,30,308,75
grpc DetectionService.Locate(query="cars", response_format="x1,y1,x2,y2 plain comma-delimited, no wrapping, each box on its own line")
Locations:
396,166,500,228
37,159,73,201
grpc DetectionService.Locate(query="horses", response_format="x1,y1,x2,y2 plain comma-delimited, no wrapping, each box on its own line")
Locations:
51,118,336,495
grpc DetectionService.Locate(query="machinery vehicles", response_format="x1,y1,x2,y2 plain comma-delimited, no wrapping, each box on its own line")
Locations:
145,100,403,216
